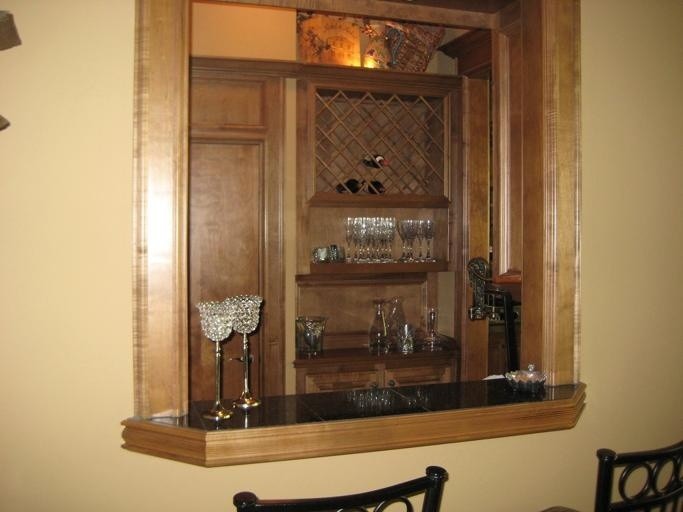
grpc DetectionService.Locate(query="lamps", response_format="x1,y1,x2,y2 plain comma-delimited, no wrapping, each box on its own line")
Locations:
0,11,23,131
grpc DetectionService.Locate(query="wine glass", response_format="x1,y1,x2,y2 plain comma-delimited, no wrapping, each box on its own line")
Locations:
345,390,427,414
367,294,441,356
341,216,438,266
194,300,236,422
224,292,263,409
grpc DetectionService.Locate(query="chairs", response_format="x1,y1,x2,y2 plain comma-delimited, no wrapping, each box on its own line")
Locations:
540,440,683,512
233,465,449,512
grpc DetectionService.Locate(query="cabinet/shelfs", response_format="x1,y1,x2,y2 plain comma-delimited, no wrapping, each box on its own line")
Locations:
292,70,461,394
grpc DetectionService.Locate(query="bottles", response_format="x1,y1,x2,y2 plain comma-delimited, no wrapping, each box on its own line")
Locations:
360,180,387,195
360,151,389,169
336,178,363,194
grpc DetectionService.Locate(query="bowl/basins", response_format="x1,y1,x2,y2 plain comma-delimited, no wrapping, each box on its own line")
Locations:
505,370,547,391
